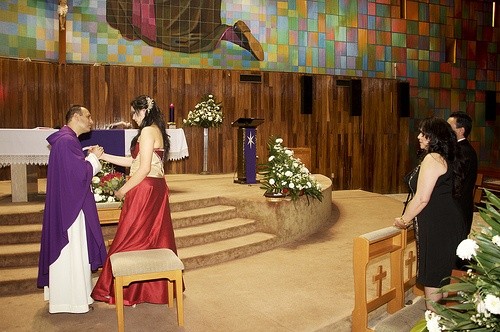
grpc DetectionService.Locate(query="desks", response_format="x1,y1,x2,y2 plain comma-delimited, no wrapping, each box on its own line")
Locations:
0,127,189,203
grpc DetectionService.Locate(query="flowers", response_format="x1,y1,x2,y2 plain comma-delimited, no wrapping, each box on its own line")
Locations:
254,134,324,206
411,189,500,332
181,94,225,129
91,159,127,209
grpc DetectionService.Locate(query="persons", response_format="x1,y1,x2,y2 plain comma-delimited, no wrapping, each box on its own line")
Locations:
448,111,478,243
88,95,186,306
38,105,108,313
395,117,459,315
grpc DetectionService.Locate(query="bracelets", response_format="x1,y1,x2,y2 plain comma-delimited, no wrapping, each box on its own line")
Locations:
401,218,407,226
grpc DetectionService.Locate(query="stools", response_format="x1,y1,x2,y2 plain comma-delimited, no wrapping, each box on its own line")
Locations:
110,247,184,332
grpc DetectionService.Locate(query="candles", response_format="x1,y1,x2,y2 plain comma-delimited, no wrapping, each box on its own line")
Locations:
170,104,175,122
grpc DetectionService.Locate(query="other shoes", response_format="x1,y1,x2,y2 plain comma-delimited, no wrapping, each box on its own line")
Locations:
89,306,95,311
